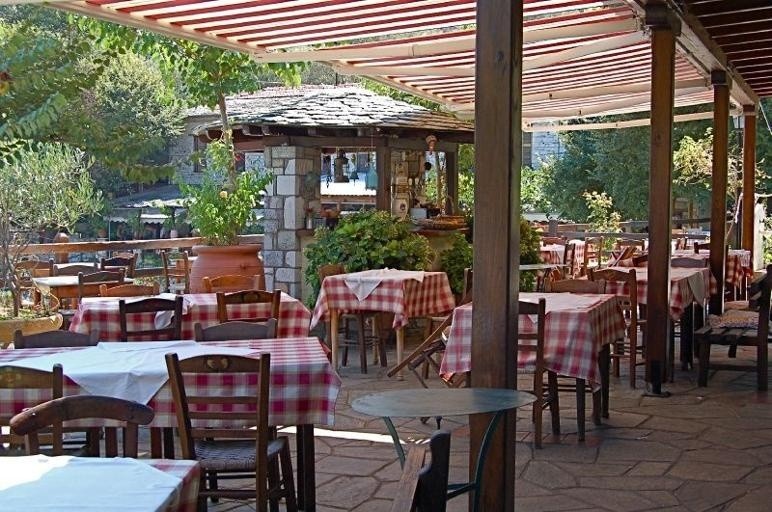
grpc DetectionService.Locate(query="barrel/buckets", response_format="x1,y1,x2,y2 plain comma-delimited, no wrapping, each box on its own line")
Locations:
190,243,265,293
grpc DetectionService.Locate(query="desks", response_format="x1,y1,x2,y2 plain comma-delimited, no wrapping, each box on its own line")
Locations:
68,287,313,337
1,451,206,512
4,337,343,512
346,383,540,511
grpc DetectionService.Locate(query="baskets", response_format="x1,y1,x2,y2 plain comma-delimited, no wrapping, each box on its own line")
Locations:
1,271,60,313
423,196,464,230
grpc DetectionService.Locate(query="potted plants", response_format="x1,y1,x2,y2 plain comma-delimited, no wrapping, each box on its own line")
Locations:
0,134,108,351
160,130,281,293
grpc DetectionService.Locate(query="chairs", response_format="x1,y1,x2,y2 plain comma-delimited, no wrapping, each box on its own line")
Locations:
213,288,282,339
1,362,67,456
158,347,296,512
5,393,156,461
201,273,262,292
13,246,198,302
11,327,101,348
189,316,280,343
112,295,184,341
310,225,771,451
